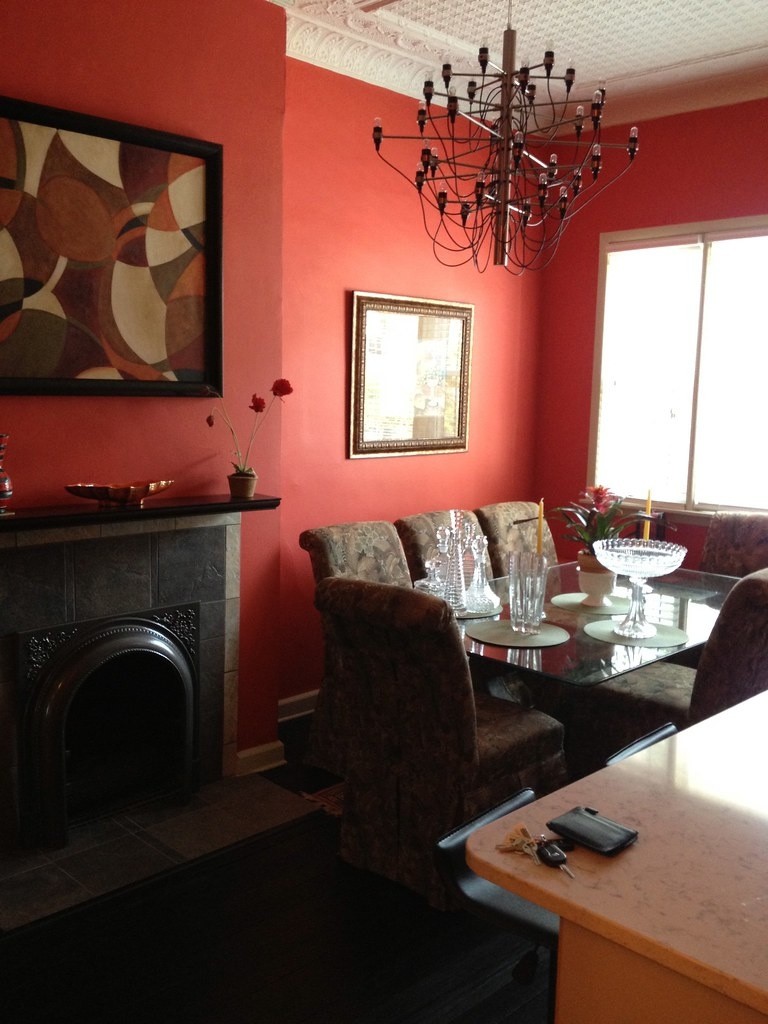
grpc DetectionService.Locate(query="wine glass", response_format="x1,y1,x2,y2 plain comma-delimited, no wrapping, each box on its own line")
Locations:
593,539,687,640
624,645,644,670
577,566,617,606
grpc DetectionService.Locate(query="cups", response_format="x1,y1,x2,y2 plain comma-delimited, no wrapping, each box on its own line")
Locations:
508,548,550,636
505,646,542,673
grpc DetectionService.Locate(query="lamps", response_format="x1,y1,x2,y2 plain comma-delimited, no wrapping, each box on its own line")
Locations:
371,0,643,279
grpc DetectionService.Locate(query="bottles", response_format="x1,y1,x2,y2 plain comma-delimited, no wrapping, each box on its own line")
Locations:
0,432,13,512
413,510,501,616
455,612,502,656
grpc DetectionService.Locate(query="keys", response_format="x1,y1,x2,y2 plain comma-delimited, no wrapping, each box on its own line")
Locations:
495,834,577,881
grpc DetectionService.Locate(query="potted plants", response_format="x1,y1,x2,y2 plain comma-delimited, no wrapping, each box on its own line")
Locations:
298,495,767,902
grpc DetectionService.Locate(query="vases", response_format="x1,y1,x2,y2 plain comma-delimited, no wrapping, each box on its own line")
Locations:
205,377,295,495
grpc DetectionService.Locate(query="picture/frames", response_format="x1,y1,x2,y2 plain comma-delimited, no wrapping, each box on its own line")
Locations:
0,95,226,401
344,288,475,461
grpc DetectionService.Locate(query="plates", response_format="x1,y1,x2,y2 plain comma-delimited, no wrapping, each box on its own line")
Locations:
64,478,176,511
